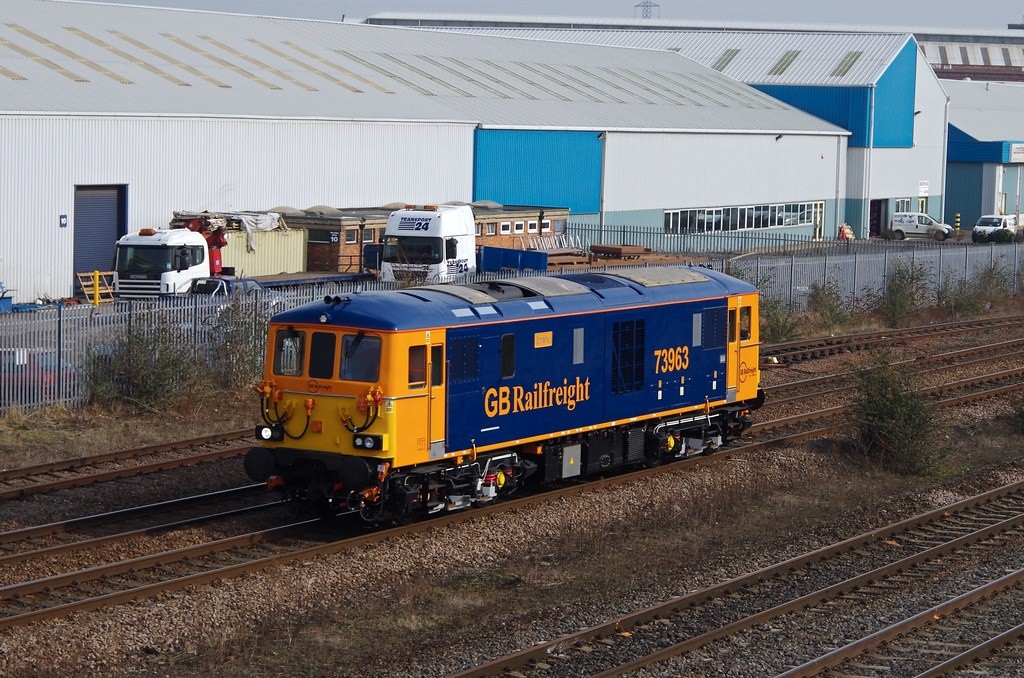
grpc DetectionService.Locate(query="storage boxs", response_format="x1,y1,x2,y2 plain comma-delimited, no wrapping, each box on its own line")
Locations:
0,297,12,314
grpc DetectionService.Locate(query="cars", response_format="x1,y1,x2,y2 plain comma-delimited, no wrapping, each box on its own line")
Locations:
158,273,290,317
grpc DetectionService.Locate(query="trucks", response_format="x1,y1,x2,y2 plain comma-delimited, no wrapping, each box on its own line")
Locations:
381,202,478,287
110,210,308,314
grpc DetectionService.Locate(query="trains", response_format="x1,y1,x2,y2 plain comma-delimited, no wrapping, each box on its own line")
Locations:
243,264,761,530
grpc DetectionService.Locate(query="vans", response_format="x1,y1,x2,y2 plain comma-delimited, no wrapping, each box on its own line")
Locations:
971,215,1018,244
890,212,955,242
